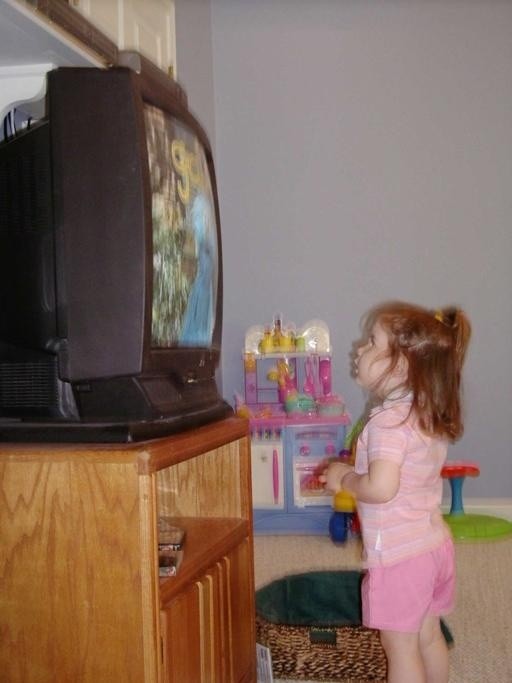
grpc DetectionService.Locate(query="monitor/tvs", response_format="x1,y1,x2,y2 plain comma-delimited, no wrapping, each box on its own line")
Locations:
0,66,233,445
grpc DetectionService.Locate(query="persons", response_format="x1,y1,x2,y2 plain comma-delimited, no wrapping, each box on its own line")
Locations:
319,301,471,683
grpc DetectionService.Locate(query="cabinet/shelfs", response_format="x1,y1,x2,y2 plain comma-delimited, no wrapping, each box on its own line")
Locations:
0,417,262,683
